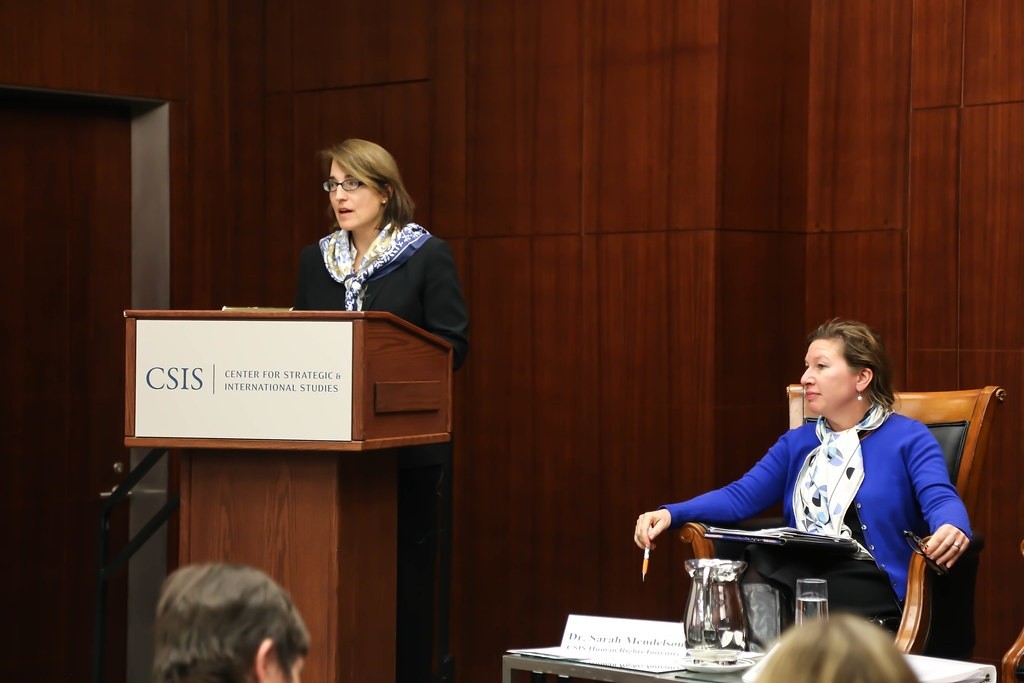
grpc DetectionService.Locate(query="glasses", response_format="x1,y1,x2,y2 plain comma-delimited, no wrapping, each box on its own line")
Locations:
903,530,949,576
322,179,387,192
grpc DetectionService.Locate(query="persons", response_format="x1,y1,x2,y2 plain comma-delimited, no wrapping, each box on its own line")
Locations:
750,615,921,683
149,560,309,683
295,137,469,683
632,319,971,652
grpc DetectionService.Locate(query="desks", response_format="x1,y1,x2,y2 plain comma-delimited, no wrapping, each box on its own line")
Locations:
501,638,995,683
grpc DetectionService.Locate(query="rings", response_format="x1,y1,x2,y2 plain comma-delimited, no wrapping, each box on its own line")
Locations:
953,542,961,549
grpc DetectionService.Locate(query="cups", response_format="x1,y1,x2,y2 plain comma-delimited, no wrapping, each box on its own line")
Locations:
795,579,829,627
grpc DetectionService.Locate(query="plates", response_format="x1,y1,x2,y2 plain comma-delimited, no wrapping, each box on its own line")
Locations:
681,658,755,673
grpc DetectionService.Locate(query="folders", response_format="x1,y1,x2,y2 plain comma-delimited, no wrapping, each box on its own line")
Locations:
703,532,860,547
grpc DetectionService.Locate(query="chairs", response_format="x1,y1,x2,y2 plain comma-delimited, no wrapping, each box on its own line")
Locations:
682,386,1004,654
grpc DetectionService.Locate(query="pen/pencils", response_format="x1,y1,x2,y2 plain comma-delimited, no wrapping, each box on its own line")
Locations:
641,524,653,583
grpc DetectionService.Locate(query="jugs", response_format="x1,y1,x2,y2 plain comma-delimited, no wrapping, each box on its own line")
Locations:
684,559,748,665
